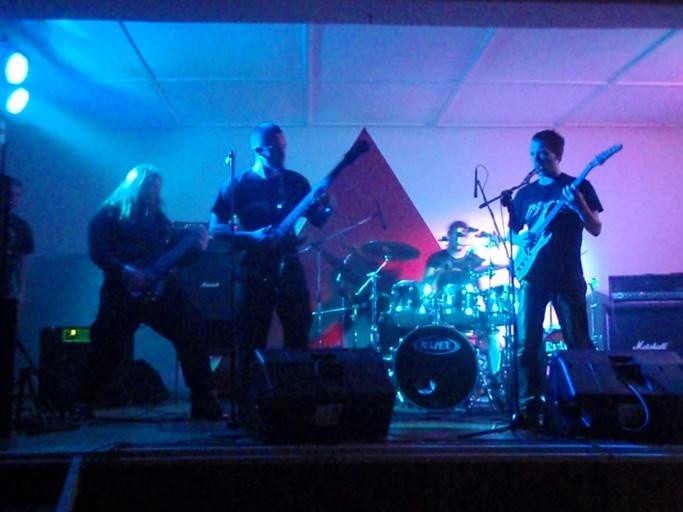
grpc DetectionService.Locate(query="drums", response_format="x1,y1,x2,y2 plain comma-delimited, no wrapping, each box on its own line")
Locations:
336,253,399,313
392,324,479,412
340,305,398,352
436,283,487,326
474,288,516,325
390,280,440,328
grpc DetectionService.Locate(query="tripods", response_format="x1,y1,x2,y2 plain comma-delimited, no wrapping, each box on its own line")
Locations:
459,180,549,440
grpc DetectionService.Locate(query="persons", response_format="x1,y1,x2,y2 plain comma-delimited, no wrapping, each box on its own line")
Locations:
420,221,506,375
89,164,230,421
508,129,603,399
0,174,35,434
208,122,338,423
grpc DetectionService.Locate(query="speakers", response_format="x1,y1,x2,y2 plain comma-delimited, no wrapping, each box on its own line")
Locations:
37,326,135,415
243,347,397,444
548,349,683,446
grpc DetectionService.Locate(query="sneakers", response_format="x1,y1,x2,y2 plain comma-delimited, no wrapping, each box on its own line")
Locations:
522,413,538,425
191,401,224,419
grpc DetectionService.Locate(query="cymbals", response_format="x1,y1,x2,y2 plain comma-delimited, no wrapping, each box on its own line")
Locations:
306,296,341,339
361,241,419,262
479,263,506,275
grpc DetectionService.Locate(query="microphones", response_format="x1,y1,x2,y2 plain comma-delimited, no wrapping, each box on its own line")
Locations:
376,201,387,230
474,169,478,197
225,150,233,165
524,166,544,181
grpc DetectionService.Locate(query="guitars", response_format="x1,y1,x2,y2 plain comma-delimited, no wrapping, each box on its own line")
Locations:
124,223,208,313
512,144,622,285
219,140,370,284
